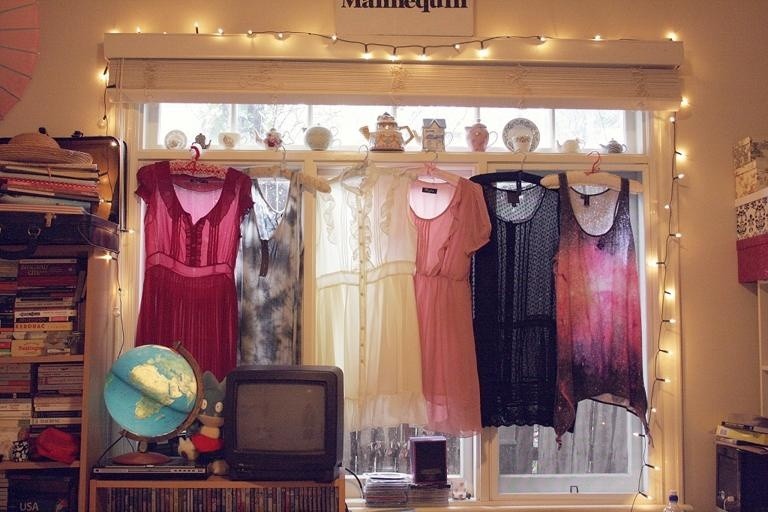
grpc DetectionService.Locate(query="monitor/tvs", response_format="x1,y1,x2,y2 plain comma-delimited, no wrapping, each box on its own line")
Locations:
223,366,346,483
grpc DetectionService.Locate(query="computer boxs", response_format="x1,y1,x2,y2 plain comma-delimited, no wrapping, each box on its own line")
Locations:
712,443,768,512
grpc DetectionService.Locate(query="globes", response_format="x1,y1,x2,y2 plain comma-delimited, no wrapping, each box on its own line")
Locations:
103,341,205,465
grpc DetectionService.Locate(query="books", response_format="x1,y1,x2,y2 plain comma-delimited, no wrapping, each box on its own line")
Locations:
716,421,768,447
0,470,9,512
95,487,339,512
0,160,100,461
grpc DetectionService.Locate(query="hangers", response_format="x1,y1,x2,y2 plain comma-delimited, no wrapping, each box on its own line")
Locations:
137,145,647,196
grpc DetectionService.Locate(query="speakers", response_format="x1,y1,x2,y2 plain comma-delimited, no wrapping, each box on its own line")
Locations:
409,436,448,484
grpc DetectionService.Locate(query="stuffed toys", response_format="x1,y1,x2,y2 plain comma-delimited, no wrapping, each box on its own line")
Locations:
178,371,231,475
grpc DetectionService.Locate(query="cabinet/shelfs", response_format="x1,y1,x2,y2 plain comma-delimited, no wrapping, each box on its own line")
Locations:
90,469,344,512
756,282,767,420
1,203,118,511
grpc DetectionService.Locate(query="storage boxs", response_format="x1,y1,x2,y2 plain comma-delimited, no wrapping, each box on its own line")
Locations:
730,138,768,282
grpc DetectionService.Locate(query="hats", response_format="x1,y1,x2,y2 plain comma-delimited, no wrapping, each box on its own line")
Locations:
1,133,93,166
33,428,79,465
